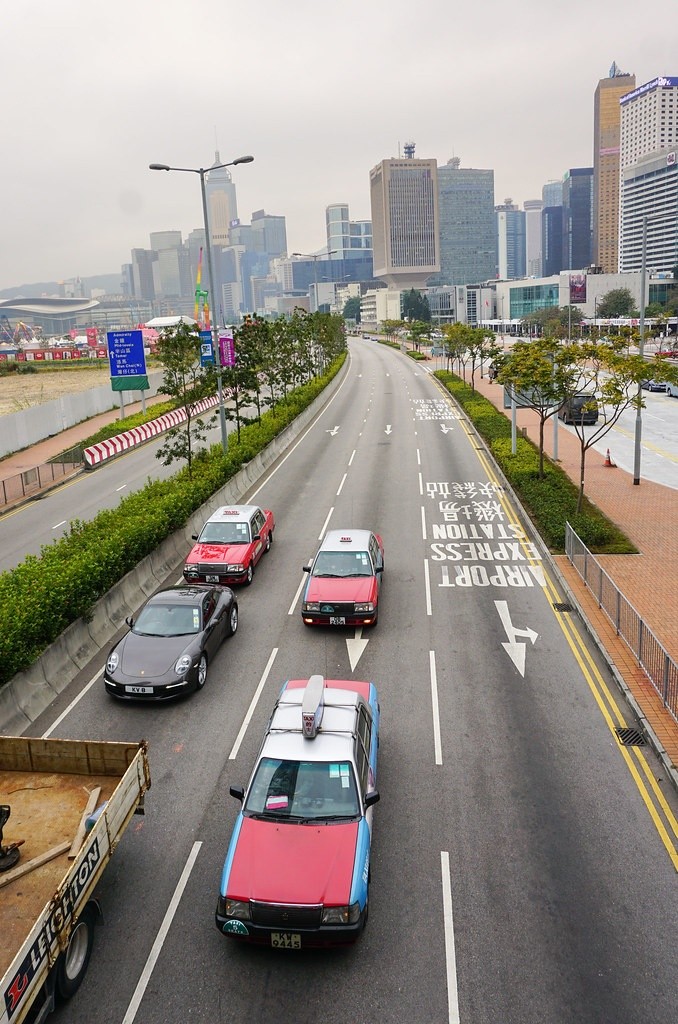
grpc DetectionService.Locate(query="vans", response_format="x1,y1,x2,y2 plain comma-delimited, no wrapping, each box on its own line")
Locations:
559,389,599,424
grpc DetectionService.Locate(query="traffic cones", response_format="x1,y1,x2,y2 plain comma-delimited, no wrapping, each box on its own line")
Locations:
602,448,614,467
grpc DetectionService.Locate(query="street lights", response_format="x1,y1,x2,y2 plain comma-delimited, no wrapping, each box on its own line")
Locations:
149,155,257,455
291,250,338,315
322,275,351,313
595,293,603,327
552,284,571,350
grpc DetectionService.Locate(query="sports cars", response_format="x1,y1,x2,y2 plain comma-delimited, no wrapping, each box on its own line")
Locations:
103,580,240,711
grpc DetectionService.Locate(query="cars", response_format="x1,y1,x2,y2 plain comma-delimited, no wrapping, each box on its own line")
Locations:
655,346,678,360
183,504,275,588
640,376,667,392
362,333,370,339
215,674,381,952
301,529,386,631
371,336,378,340
665,380,678,397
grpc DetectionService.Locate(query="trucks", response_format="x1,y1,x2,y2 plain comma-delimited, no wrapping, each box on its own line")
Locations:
0,734,154,1024
